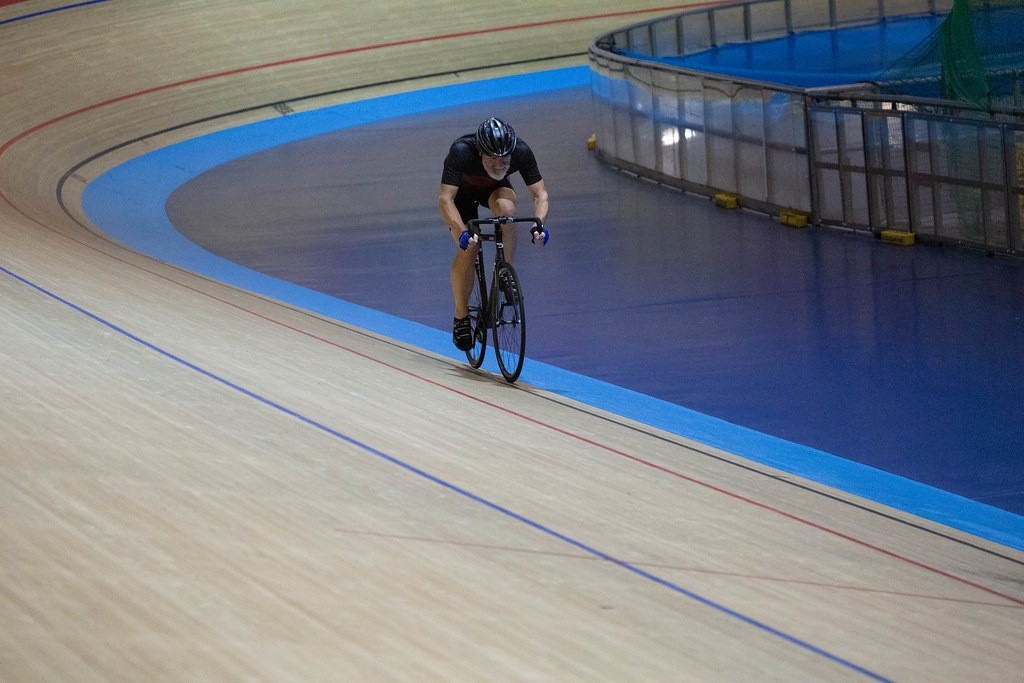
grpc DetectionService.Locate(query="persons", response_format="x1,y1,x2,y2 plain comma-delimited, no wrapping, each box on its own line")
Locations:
438,115,549,352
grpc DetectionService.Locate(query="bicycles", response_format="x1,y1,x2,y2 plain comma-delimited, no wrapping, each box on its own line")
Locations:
464,217,544,384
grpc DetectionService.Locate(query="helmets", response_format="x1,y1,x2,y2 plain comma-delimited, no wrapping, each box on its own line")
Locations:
476,117,516,160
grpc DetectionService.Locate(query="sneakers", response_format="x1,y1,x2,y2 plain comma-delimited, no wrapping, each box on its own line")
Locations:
453,314,472,351
498,264,519,301
